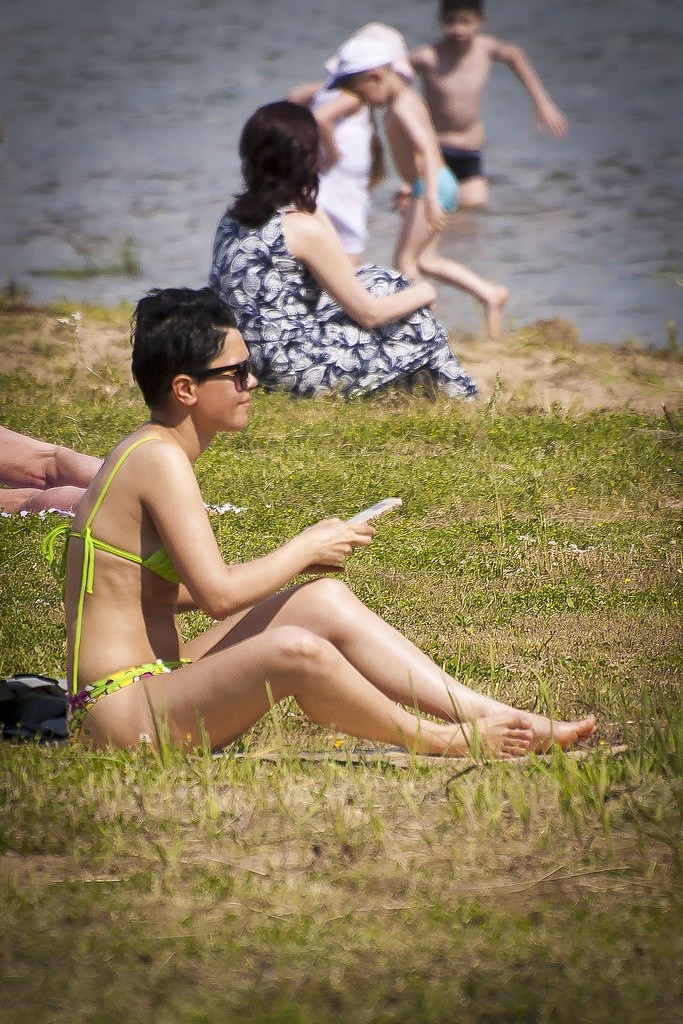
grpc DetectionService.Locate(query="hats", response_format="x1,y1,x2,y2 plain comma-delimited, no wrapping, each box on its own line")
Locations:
351,21,416,79
325,36,394,89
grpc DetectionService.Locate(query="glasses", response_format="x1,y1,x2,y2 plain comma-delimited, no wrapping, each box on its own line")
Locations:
191,354,253,382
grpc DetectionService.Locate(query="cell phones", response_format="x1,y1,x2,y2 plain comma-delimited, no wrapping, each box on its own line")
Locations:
347,498,403,525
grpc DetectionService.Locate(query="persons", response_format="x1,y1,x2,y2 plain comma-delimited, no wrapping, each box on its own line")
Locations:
0,425,105,514
65,288,599,760
288,0,570,339
209,101,480,401
